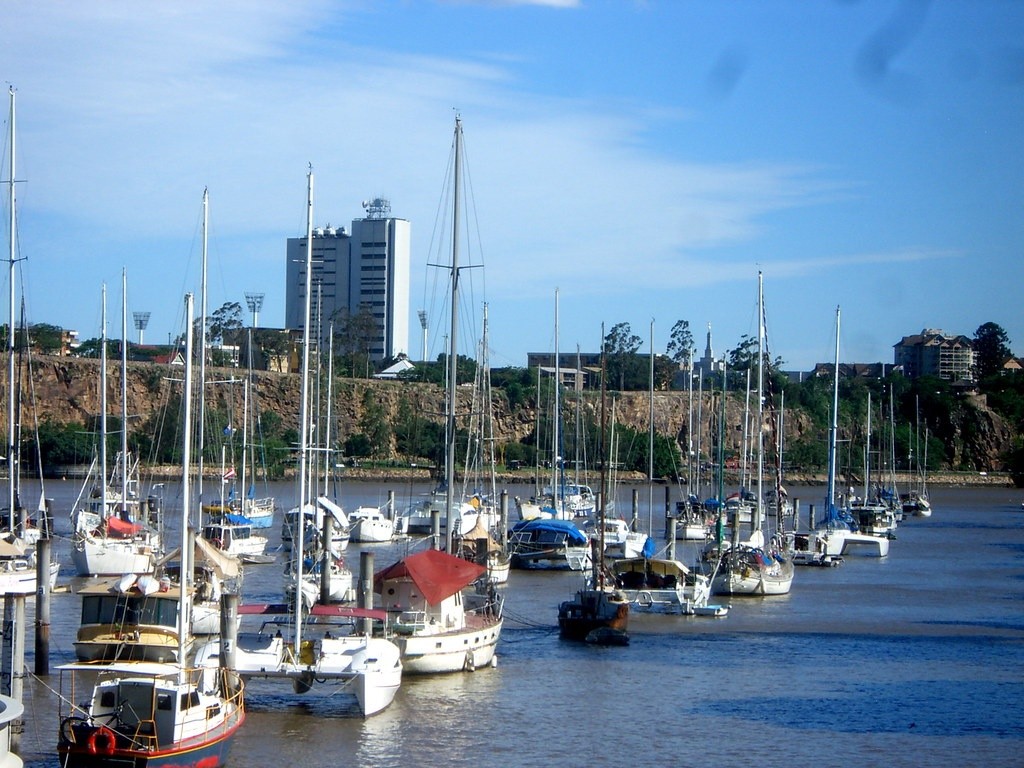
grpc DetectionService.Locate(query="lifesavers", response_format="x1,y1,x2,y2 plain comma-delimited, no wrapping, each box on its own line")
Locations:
87,728,117,756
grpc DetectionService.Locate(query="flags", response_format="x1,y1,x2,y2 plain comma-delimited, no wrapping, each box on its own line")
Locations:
223,468,236,480
224,425,236,436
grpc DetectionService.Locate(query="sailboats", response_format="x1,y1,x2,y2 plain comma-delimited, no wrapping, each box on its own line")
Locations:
0,82,936,768
